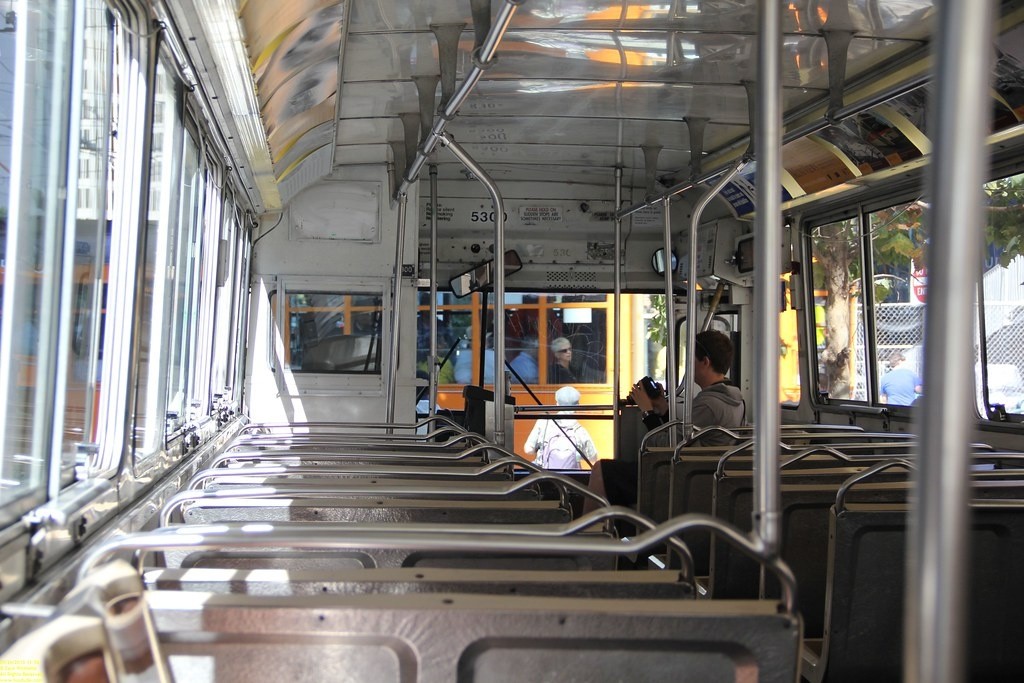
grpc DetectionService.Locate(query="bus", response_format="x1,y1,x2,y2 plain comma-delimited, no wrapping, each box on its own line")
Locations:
0,244,859,470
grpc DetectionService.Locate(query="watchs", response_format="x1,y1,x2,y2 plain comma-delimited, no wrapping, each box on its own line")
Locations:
641,410,653,421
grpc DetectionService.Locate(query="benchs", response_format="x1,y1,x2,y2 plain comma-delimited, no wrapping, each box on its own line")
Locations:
0,416,1024,683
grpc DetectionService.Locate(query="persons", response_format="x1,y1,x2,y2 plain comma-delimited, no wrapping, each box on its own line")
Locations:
630,330,747,448
879,353,923,406
524,386,597,470
416,369,444,442
416,292,579,384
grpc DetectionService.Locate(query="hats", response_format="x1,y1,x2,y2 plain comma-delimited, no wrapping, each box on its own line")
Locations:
555,387,580,406
466,325,492,339
1009,305,1024,319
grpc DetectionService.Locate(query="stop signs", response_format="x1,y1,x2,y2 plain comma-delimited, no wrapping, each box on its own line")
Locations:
910,258,929,307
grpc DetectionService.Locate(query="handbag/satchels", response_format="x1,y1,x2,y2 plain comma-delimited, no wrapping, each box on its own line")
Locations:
535,448,544,467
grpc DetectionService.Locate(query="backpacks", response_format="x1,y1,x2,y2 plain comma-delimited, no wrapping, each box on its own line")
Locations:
544,419,581,469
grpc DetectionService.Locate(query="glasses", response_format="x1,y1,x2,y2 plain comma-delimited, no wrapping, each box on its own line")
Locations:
555,347,573,353
